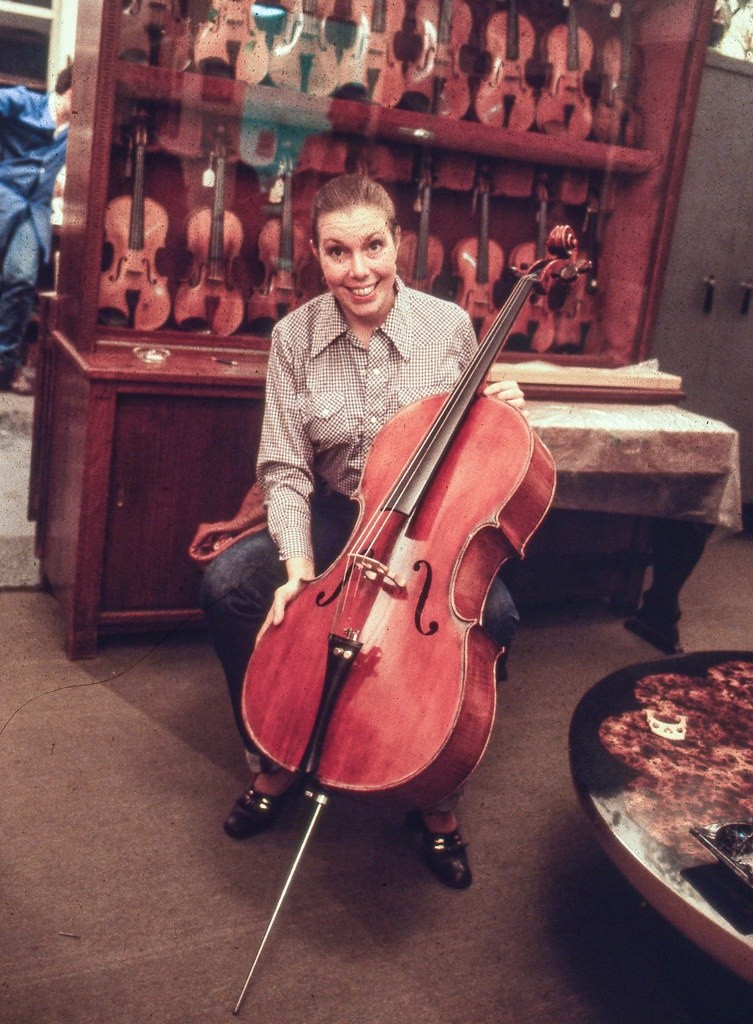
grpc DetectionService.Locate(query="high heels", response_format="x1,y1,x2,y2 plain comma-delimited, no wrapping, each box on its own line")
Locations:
225,770,306,837
402,804,472,890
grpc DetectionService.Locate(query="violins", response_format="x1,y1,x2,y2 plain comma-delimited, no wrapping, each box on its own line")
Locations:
97,0,644,355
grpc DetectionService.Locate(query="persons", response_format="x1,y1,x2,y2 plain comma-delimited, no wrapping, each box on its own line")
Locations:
0,65,76,395
199,174,530,886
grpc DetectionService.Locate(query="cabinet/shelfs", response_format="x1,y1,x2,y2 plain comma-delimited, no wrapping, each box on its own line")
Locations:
651,45,753,506
496,402,747,661
29,0,714,662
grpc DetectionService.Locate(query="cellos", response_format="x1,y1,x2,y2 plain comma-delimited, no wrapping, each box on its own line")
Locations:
228,220,596,1016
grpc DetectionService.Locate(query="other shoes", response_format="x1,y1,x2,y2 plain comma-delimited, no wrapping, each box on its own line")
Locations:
7,374,34,395
18,365,35,383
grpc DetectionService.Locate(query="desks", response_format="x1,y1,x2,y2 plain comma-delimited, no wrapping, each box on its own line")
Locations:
568,651,752,982
